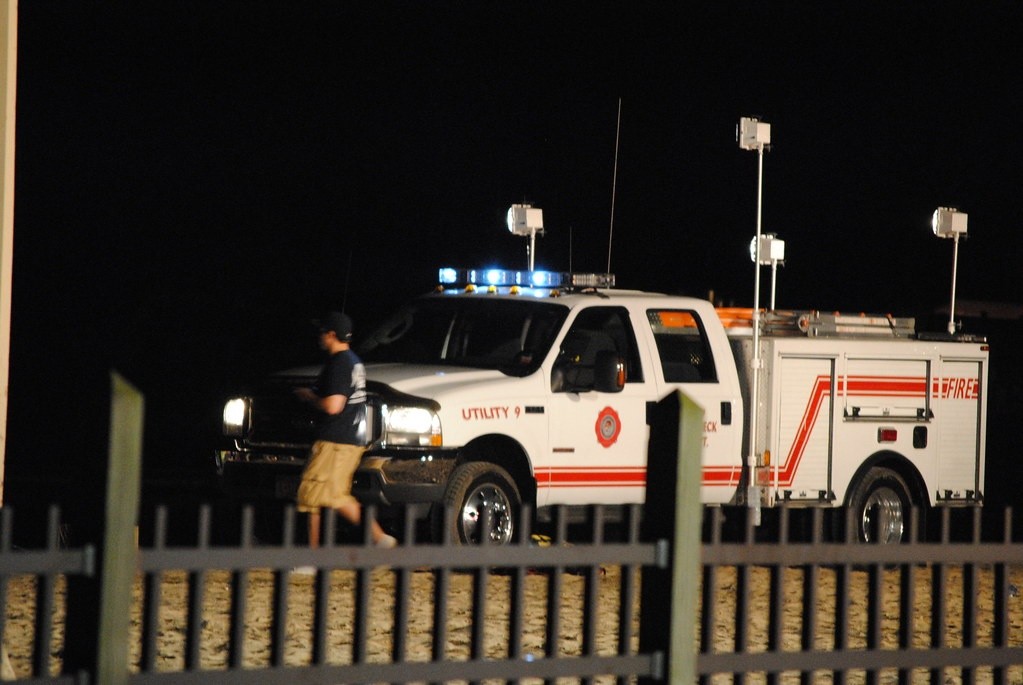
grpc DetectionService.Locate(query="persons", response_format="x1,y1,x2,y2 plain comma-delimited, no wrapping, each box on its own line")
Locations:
285,313,397,578
513,329,577,391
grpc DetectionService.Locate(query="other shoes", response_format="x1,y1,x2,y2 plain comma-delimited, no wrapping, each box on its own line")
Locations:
376,535,396,549
290,566,316,575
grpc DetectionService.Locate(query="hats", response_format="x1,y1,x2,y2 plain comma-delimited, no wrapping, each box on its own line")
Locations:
312,312,352,341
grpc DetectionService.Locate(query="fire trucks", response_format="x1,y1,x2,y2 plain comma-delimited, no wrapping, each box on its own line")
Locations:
214,269,992,575
